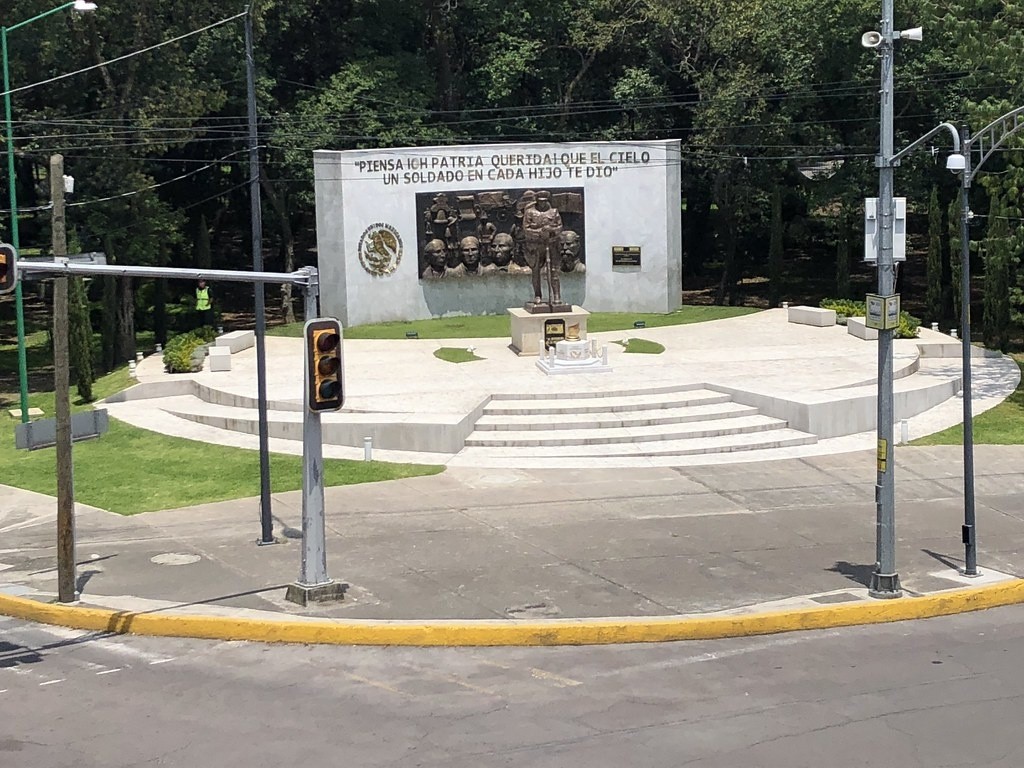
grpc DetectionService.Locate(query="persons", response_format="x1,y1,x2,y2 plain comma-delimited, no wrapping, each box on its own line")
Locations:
521,190,562,306
194,279,223,334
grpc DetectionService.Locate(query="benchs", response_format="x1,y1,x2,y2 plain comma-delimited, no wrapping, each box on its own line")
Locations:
215,329,256,354
787,305,836,328
208,346,232,373
847,316,878,340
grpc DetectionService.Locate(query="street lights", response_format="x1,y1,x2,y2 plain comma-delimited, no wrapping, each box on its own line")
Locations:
0,0,98,425
865,120,969,600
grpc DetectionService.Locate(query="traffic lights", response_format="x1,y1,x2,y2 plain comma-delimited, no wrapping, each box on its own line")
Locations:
304,317,346,414
0,243,17,295
